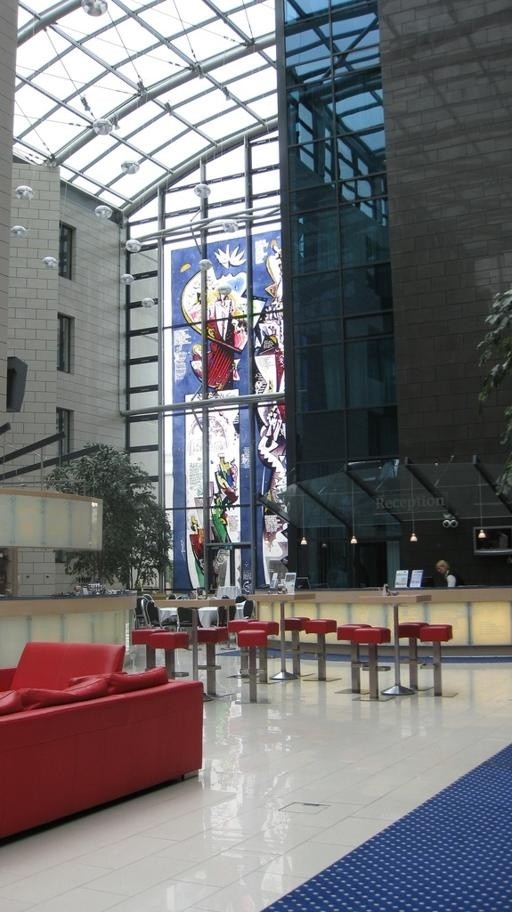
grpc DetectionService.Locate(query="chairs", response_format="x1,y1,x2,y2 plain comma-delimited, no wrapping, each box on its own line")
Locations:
0,640,203,848
135,586,254,651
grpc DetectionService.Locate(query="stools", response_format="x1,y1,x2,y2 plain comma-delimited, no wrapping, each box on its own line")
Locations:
337,622,452,700
237,629,266,703
134,626,228,698
228,619,337,682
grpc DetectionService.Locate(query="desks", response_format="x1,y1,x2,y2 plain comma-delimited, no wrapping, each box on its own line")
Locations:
247,593,316,680
360,595,432,695
154,599,235,702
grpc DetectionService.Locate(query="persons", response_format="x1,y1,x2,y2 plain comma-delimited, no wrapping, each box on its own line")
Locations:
435,560,463,587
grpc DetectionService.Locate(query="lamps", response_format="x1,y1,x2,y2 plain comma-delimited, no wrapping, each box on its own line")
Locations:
350,469,358,545
80,0,109,17
10,146,68,270
478,469,486,539
93,112,159,308
193,136,240,296
298,465,308,546
409,469,417,542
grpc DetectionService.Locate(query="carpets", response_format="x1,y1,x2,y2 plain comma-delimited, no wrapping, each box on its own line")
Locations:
258,742,512,912
